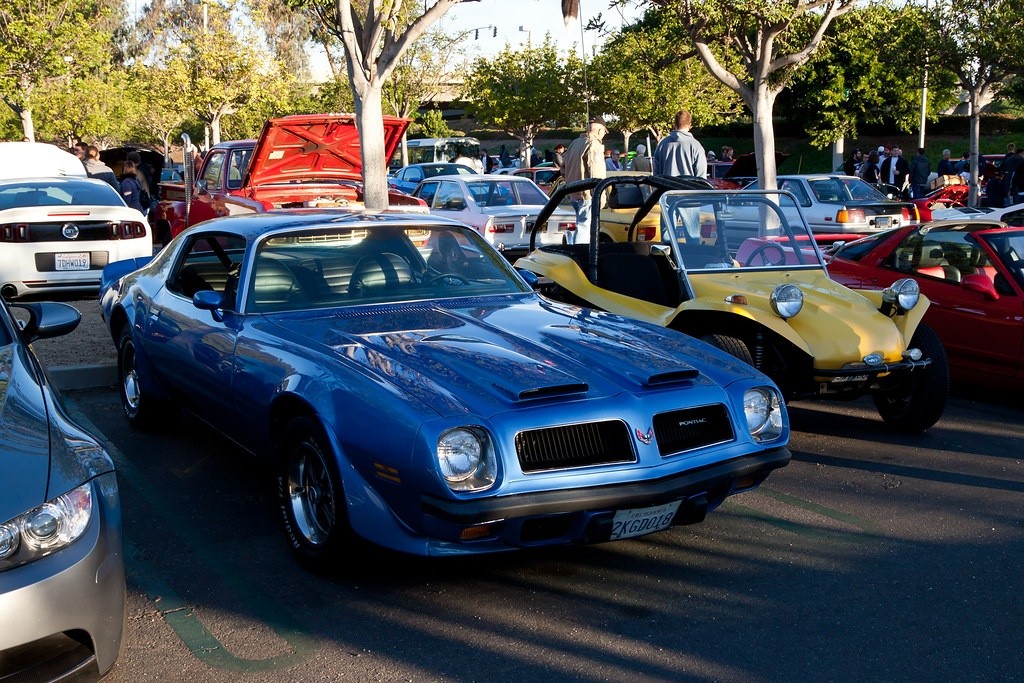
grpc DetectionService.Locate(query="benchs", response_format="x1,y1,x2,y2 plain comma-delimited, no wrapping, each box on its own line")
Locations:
543,243,666,295
181,257,355,306
893,243,971,275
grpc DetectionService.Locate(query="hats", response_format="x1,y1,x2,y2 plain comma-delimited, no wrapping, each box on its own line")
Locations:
590,116,609,134
851,144,892,154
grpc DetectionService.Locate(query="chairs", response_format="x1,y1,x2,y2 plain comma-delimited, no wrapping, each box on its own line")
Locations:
348,252,421,298
234,258,309,311
971,239,1000,286
71,191,104,203
598,254,666,308
667,242,728,309
13,192,40,206
913,242,946,280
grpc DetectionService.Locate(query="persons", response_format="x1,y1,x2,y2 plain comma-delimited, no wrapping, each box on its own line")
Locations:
846,141,1024,209
81,146,120,195
604,149,623,172
722,147,737,162
706,151,718,162
21,136,30,143
560,116,612,227
629,145,653,173
73,142,89,163
480,141,567,180
653,111,708,181
721,146,730,157
127,151,159,221
118,160,149,216
189,145,243,180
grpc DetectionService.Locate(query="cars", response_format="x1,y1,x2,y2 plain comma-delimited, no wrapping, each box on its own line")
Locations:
94,142,176,206
726,215,1024,392
382,138,561,196
0,293,132,682
704,150,792,193
0,140,156,298
565,174,719,242
154,114,429,244
410,175,576,256
97,206,792,578
728,174,920,235
868,154,1024,231
510,171,952,442
